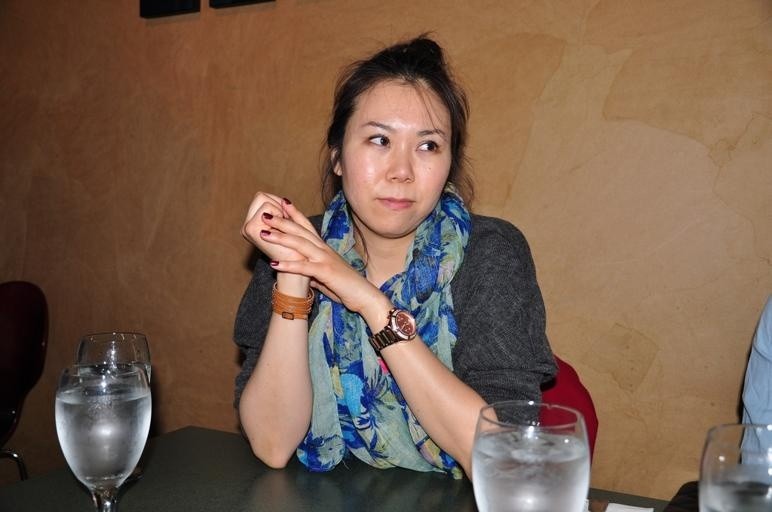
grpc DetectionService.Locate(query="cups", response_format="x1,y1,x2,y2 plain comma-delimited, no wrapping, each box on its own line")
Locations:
472,400,591,512
74,330,149,366
697,424,772,511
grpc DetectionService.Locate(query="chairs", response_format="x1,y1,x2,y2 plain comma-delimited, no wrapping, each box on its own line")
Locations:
0,281,50,483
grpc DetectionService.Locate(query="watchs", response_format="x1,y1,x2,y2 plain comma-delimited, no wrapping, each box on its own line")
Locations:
368,307,416,352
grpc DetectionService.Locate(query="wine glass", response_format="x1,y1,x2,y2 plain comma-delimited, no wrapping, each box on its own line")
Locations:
54,361,153,512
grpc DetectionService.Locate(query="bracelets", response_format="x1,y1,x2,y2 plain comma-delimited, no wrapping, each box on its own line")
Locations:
271,281,314,319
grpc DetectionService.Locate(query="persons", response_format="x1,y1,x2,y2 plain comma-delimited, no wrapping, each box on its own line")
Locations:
233,27,561,487
